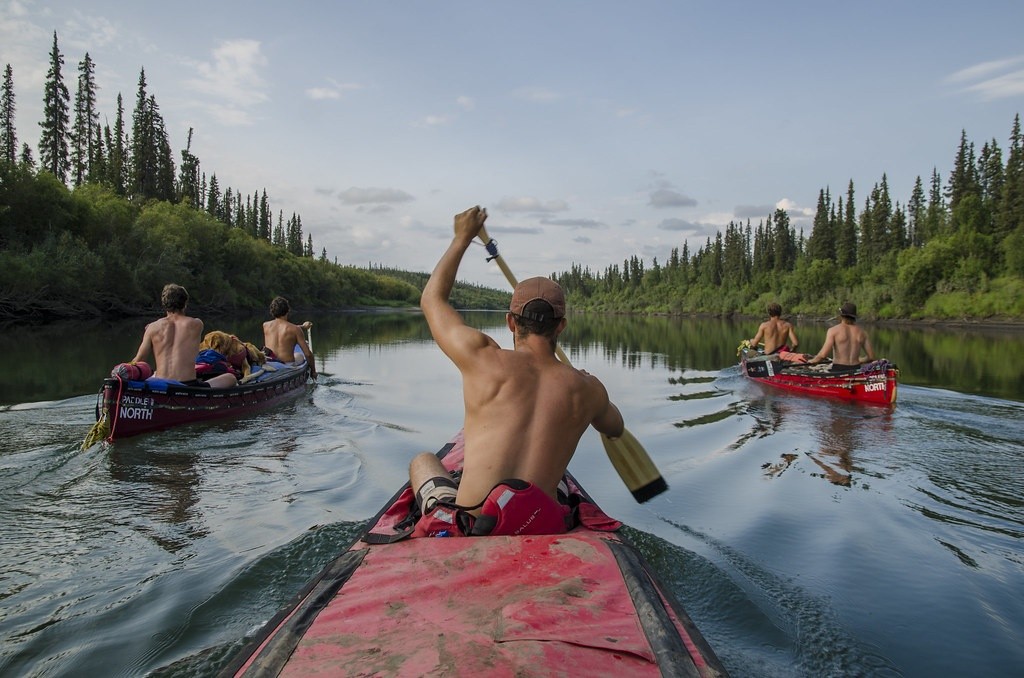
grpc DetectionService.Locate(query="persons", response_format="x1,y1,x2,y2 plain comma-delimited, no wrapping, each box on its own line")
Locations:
409,204,624,520
807,303,874,372
750,302,799,356
132,283,238,388
262,298,317,382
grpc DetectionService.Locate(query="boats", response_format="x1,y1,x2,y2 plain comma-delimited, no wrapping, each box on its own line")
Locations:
94,341,311,441
223,426,732,678
740,339,900,405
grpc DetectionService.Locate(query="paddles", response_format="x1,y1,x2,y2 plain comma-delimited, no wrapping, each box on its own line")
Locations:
745,359,833,378
306,327,319,384
476,221,670,507
757,342,765,346
236,362,279,387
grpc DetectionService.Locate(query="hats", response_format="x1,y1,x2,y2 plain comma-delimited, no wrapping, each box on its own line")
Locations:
510,276,565,321
837,303,860,318
